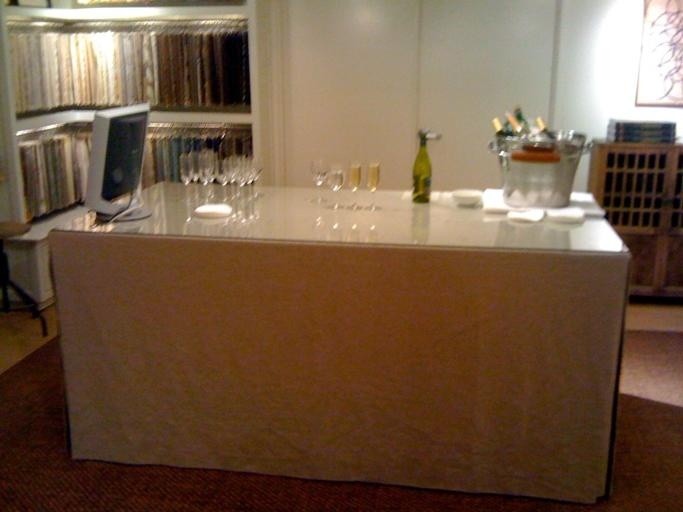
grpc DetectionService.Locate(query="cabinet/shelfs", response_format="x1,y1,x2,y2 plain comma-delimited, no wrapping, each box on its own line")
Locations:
588,139,683,298
0,7,263,315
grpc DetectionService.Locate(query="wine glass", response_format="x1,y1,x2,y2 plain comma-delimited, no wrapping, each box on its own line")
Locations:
368,164,382,195
310,159,328,199
348,163,365,201
178,150,263,221
329,167,345,198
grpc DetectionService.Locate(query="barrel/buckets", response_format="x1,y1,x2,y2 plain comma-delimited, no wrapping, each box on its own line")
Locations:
489,132,594,207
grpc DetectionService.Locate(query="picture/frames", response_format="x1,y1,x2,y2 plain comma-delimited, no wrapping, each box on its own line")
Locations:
633,1,682,109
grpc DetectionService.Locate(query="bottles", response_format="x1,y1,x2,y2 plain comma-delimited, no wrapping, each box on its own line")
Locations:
412,129,442,206
493,107,563,142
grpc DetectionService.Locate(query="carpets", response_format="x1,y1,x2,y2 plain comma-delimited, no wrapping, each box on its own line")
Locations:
1,330,683,512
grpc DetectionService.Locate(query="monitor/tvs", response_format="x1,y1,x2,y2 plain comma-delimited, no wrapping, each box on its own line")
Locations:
82,103,153,223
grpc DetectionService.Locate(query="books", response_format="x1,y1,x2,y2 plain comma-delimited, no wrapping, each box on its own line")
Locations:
606,117,677,145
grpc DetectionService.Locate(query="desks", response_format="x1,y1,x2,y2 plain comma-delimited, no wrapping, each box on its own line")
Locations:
48,180,634,503
